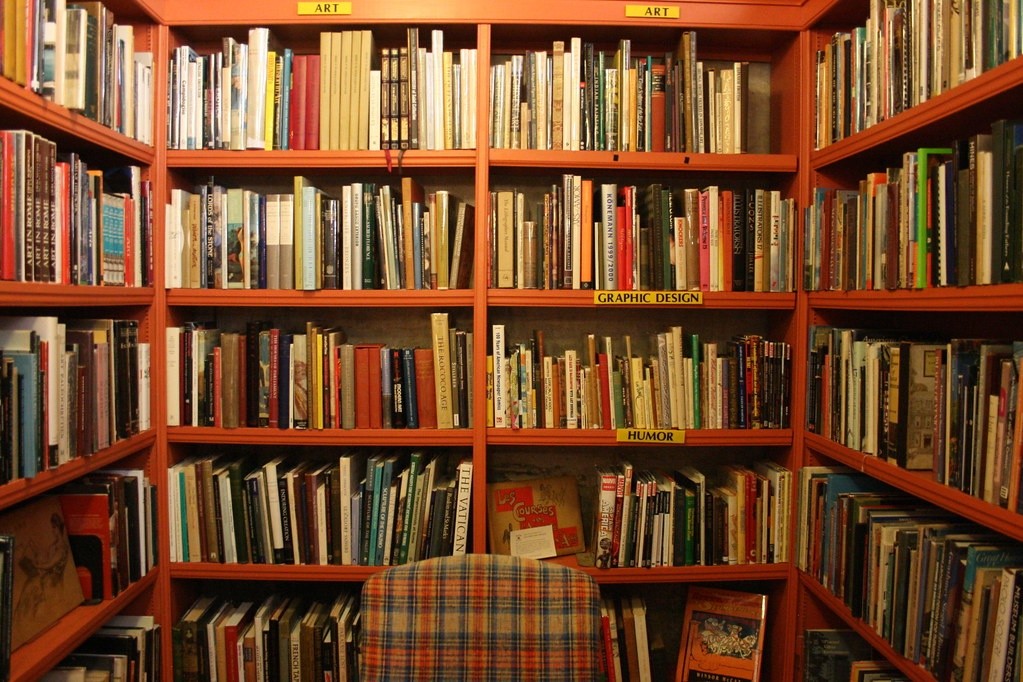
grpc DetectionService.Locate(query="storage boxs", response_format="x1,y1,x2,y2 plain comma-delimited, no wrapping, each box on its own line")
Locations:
485,462,585,563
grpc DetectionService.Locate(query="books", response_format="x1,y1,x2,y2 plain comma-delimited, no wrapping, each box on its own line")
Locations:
598,585,651,682
486,454,793,567
1,1,154,145
486,324,791,429
804,118,1023,291
793,465,1023,682
0,312,150,486
814,0,1022,153
165,175,475,290
166,448,472,566
166,313,472,429
673,584,769,682
173,580,359,681
0,469,161,682
489,174,797,292
805,325,1023,514
166,29,476,151
489,32,749,154
1,128,154,287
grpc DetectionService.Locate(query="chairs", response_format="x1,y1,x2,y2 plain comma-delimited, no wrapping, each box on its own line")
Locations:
358,554,608,682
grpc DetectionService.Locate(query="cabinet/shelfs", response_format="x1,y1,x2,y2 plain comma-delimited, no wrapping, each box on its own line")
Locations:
168,0,807,682
2,0,169,681
801,2,1023,676
